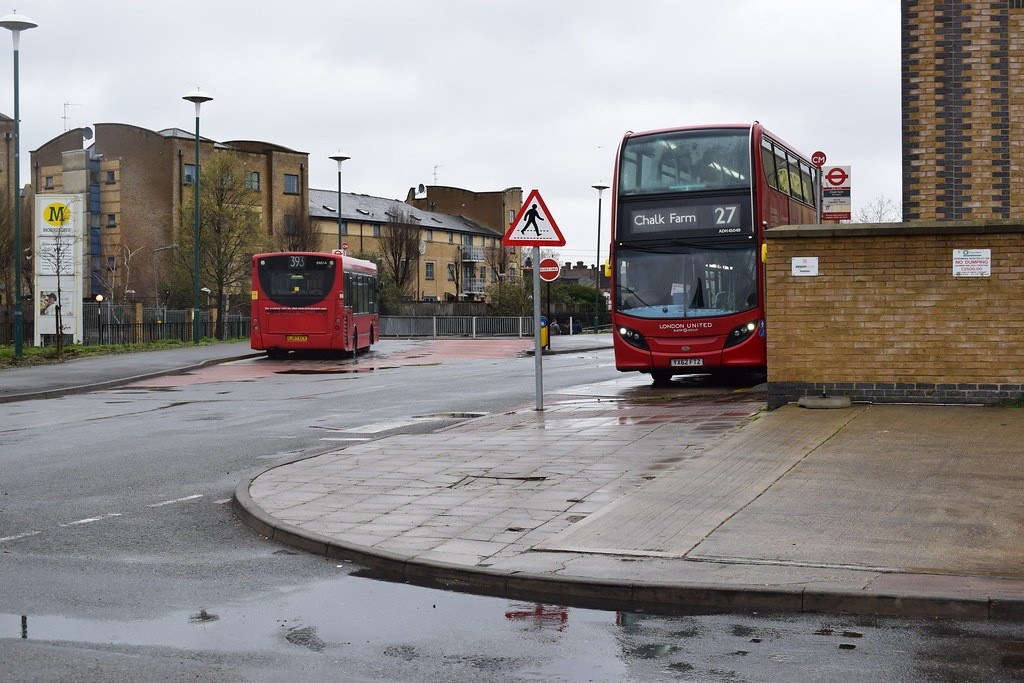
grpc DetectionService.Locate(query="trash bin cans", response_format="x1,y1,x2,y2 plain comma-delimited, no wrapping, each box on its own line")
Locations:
541,324,548,348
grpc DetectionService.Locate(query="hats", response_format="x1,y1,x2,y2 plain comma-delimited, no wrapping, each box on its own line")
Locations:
47,293,56,301
552,319,557,323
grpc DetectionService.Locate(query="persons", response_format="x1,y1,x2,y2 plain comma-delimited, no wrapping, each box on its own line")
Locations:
43,293,58,316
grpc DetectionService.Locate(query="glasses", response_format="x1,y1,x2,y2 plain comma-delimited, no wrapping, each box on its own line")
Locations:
49,297,54,299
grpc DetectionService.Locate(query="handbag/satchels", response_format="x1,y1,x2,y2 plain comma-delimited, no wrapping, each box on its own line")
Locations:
556,327,560,335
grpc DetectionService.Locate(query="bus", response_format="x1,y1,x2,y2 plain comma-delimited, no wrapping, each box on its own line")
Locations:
251,251,384,359
604,120,815,384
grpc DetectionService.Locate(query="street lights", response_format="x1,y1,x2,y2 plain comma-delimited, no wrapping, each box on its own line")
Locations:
0,13,39,354
329,154,351,250
182,91,213,342
592,184,611,334
